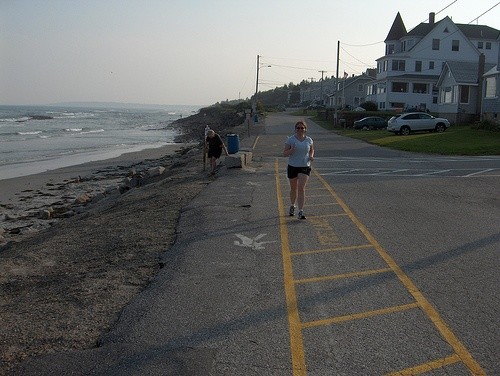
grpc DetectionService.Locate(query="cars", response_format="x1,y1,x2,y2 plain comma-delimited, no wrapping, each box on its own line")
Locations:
387,112,450,136
352,117,387,131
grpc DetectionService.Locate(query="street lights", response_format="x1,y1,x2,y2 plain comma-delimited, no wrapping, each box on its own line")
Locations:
255,65,272,97
318,71,327,80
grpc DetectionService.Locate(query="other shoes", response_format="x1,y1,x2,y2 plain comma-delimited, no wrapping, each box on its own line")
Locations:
207,172,216,177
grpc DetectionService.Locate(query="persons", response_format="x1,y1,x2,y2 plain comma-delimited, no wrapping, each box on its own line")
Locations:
283,121,315,219
205,130,228,175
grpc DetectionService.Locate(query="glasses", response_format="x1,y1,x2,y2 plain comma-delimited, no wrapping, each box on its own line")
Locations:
296,127,305,129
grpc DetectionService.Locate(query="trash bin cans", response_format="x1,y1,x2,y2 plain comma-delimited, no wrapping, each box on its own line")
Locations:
225,132,239,154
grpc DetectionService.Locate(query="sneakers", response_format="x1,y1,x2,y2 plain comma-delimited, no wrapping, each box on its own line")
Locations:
298,211,307,219
289,205,294,216
